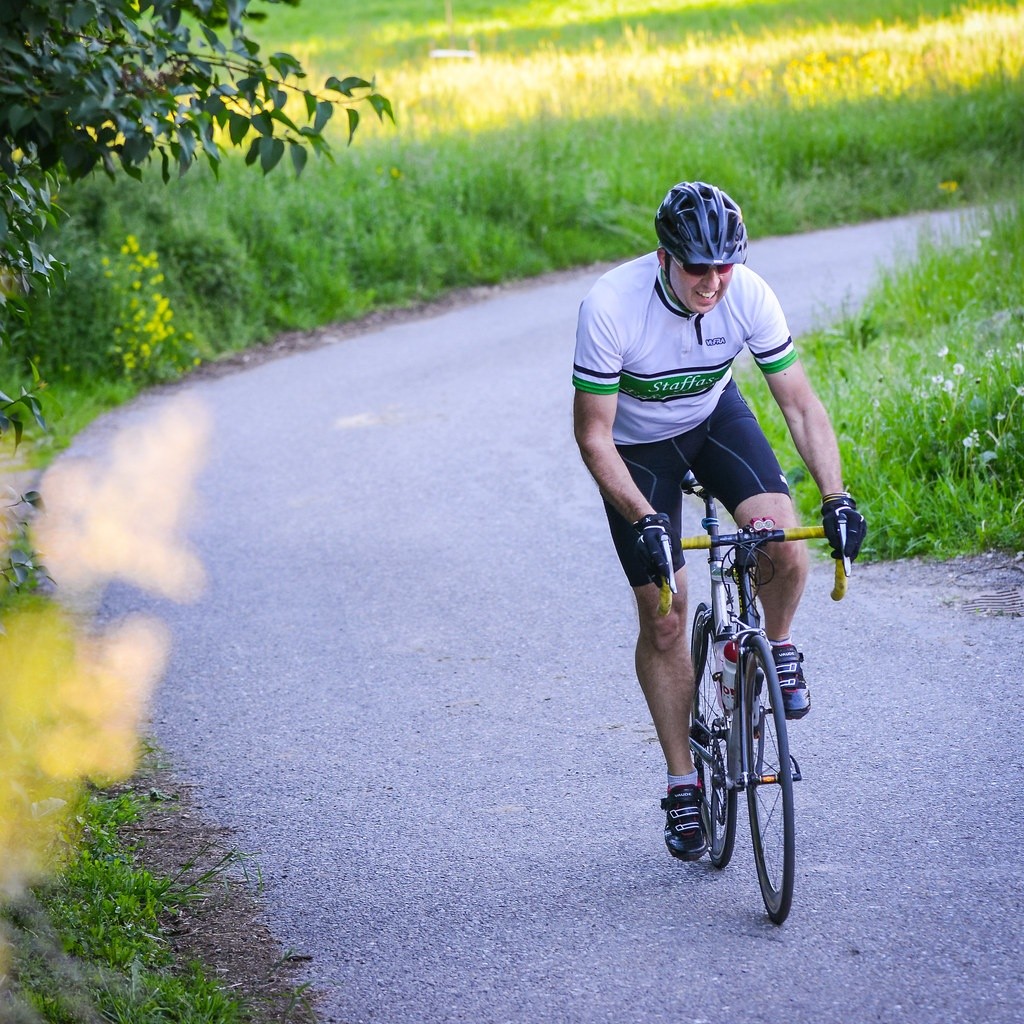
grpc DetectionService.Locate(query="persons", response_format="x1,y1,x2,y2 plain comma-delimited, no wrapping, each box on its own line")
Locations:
572,183,865,860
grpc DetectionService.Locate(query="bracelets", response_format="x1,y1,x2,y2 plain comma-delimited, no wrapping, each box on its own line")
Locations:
823,491,850,502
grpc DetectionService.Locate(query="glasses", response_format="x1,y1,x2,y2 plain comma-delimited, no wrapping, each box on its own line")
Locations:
671,256,734,275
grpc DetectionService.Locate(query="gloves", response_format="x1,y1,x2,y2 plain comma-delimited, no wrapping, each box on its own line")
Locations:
632,513,686,588
821,498,867,566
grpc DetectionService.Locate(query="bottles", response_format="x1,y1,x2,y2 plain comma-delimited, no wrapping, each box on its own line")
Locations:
720,638,739,712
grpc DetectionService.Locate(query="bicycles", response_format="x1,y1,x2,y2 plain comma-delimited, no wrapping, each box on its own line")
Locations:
655,468,849,924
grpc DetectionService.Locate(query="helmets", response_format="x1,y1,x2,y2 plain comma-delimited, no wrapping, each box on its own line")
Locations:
655,181,748,264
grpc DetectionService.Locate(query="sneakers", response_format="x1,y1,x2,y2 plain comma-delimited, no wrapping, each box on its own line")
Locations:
770,644,811,719
661,776,709,861
